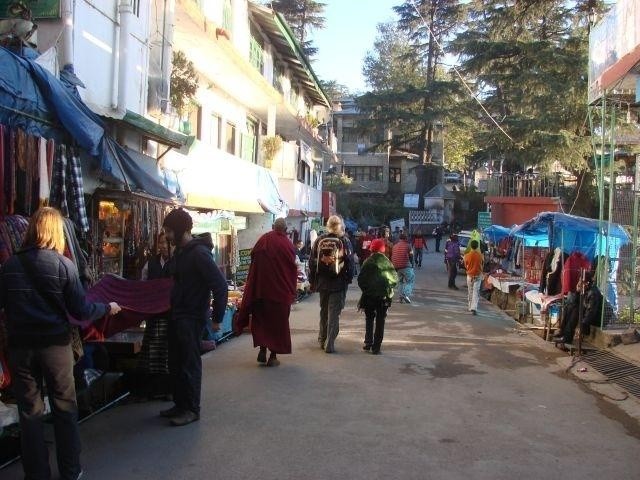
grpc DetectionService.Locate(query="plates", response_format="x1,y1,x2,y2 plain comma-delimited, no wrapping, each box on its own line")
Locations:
89,195,124,282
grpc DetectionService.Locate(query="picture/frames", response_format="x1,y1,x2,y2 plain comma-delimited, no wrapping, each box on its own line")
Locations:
525,292,564,341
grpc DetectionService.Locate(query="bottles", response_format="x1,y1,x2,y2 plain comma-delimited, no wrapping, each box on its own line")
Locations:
162,206,193,251
370,239,385,253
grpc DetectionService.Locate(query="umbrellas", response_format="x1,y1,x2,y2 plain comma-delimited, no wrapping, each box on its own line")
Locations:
554,336,572,344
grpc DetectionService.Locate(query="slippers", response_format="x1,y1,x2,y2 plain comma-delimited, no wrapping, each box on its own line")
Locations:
315,237,344,278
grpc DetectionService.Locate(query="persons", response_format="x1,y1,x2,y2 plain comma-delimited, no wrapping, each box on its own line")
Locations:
463,240,485,315
359,226,402,259
432,224,444,252
445,235,463,290
553,272,609,343
160,207,228,423
0,207,121,479
147,233,175,279
412,228,428,267
357,239,399,354
391,234,416,304
306,215,355,353
238,219,297,367
295,239,310,262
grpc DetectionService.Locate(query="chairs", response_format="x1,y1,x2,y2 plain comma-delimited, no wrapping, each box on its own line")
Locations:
169,410,200,426
402,294,411,303
256,352,266,364
267,358,280,367
160,407,175,417
